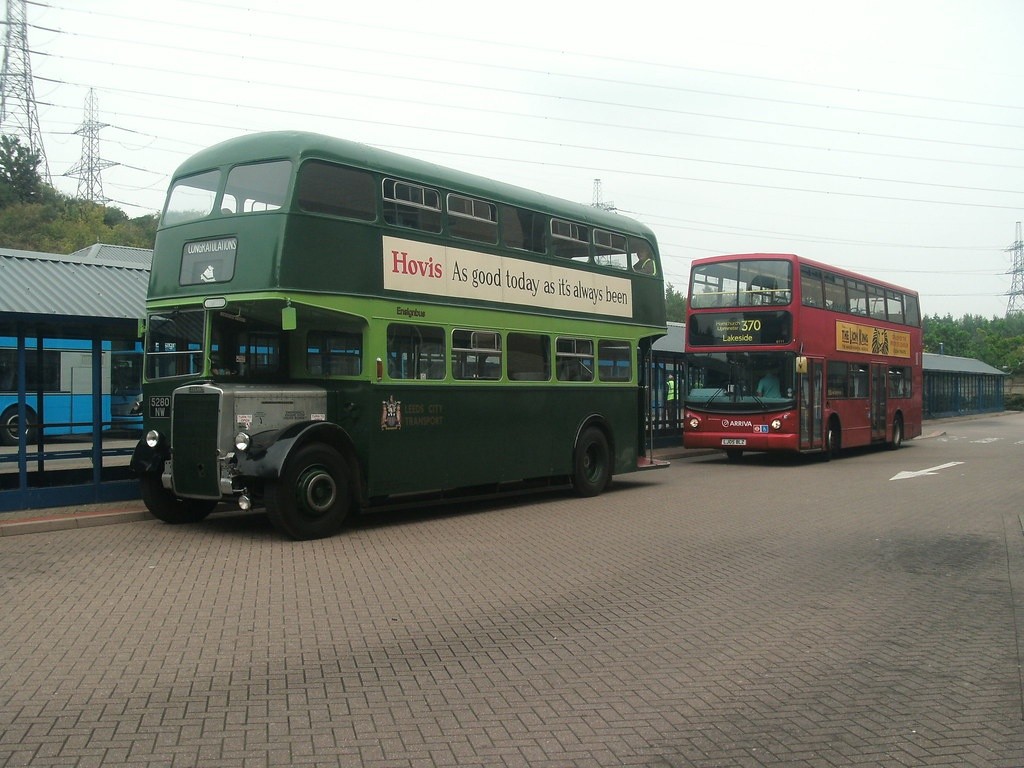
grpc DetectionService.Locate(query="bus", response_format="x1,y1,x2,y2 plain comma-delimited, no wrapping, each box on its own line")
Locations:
128,128,669,542
109,341,404,430
0,336,112,445
581,360,667,408
683,254,923,462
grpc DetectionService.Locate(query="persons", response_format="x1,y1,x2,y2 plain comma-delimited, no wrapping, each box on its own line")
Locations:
664,374,679,427
692,378,703,389
757,370,782,397
633,249,656,276
